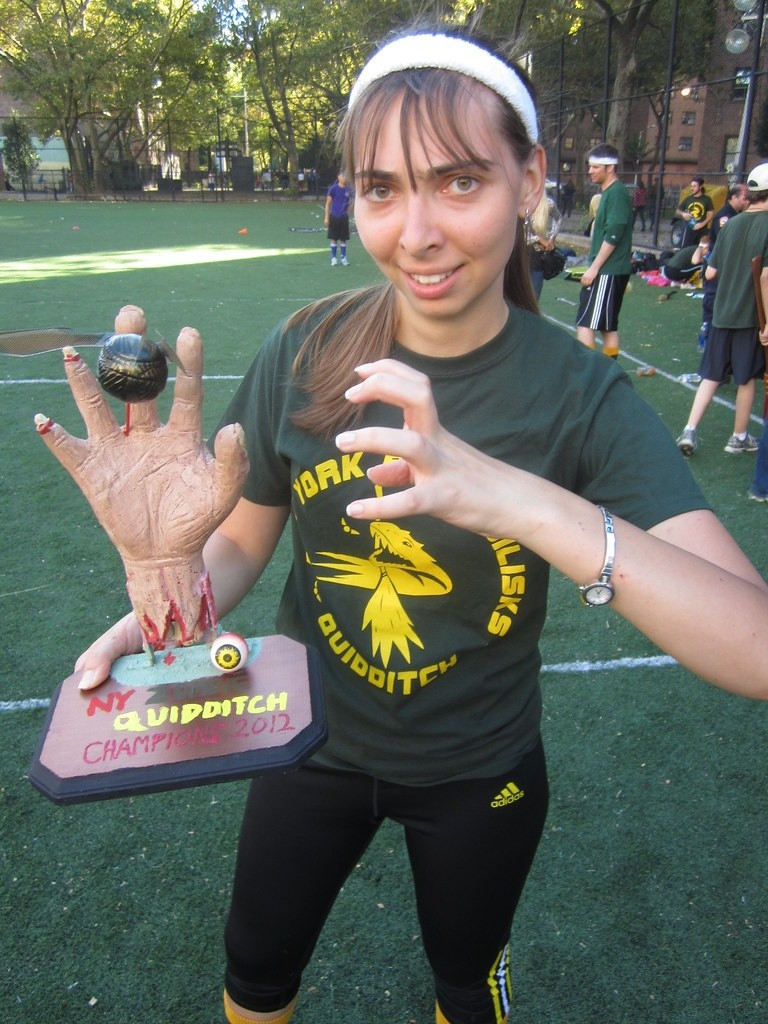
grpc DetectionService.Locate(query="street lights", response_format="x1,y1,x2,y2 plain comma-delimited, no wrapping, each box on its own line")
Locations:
724,0,768,187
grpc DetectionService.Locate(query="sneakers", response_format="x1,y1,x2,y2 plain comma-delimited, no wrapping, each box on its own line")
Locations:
675,429,697,458
724,432,760,453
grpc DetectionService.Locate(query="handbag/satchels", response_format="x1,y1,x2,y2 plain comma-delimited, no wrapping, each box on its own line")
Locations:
541,248,565,280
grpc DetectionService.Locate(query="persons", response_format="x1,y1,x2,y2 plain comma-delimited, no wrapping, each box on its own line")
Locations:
646,178,665,231
33,304,250,667
524,179,562,302
324,170,353,266
576,143,634,360
562,177,576,217
262,169,317,192
675,178,715,245
75,27,768,1024
632,181,647,231
209,170,214,191
664,236,711,289
676,163,768,457
701,184,750,330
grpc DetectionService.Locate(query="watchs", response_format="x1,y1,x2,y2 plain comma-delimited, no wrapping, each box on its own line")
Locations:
579,504,617,606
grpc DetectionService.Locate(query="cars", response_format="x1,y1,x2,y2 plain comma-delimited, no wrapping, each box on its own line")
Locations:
544,178,562,190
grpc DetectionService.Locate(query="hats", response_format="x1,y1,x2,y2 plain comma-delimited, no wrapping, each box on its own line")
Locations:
747,163,768,191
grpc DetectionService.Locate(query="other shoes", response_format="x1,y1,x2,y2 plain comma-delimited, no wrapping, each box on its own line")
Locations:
680,282,692,289
671,281,681,286
746,489,768,503
342,258,349,266
332,257,338,266
